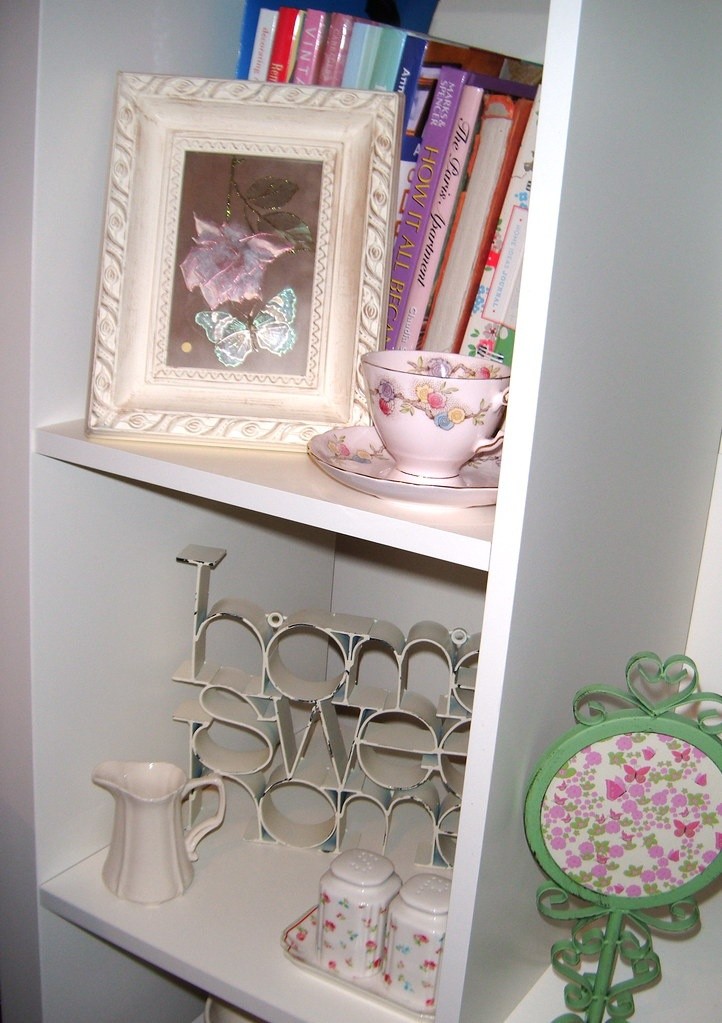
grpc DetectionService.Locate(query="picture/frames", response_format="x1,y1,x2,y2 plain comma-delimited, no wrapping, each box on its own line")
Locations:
88,72,405,457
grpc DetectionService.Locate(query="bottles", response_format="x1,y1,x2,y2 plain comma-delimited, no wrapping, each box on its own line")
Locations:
315,850,403,977
381,872,452,1006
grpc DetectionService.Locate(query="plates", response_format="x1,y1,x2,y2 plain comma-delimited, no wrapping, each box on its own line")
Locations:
280,903,434,1023
307,425,503,508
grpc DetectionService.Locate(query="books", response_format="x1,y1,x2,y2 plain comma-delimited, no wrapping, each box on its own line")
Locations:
236,0,546,427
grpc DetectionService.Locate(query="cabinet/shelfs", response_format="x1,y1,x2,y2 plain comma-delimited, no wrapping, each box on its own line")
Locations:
0,0,722,1023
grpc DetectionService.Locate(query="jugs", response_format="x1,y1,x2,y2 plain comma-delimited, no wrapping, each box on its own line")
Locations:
91,760,226,904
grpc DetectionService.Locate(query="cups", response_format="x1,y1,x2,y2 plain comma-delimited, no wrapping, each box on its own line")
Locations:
360,351,512,481
